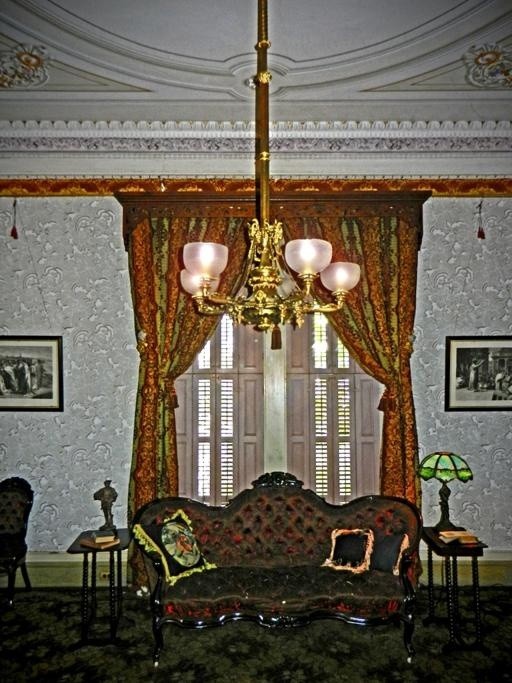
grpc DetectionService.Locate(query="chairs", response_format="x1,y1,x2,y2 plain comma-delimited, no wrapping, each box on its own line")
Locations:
0,476,34,605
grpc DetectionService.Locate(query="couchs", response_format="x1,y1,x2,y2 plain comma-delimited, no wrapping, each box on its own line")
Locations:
132,472,423,667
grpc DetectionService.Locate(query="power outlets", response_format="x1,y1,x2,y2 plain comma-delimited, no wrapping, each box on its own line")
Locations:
99,571,110,581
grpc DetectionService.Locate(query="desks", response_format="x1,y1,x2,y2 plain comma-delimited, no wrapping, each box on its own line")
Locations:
423,525,488,654
67,528,132,643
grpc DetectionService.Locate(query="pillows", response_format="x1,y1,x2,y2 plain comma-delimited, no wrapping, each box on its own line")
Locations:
370,533,410,574
320,527,375,573
133,509,217,587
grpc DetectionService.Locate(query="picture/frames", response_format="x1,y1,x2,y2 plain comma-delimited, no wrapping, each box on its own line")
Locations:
445,335,512,410
0,336,64,412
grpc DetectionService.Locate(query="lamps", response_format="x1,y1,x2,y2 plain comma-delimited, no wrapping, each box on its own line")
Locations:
418,451,473,531
180,0,361,334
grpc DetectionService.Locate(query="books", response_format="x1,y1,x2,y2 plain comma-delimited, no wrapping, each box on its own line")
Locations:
92,529,114,543
79,535,121,550
439,530,479,544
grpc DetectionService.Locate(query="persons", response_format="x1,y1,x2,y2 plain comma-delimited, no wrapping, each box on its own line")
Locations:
0,356,42,396
457,370,512,391
94,478,118,527
469,357,486,390
